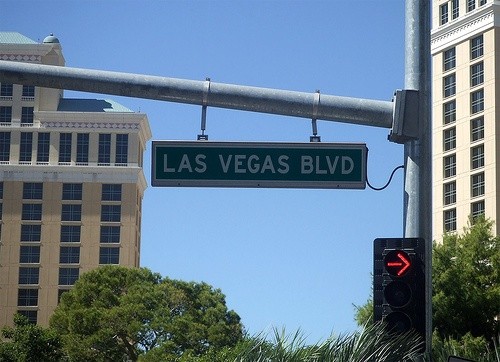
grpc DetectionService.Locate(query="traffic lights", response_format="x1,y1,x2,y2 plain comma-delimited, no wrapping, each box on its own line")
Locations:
382,247,414,336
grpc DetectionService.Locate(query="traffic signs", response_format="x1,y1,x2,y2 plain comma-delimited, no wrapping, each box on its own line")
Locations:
153,147,360,183
